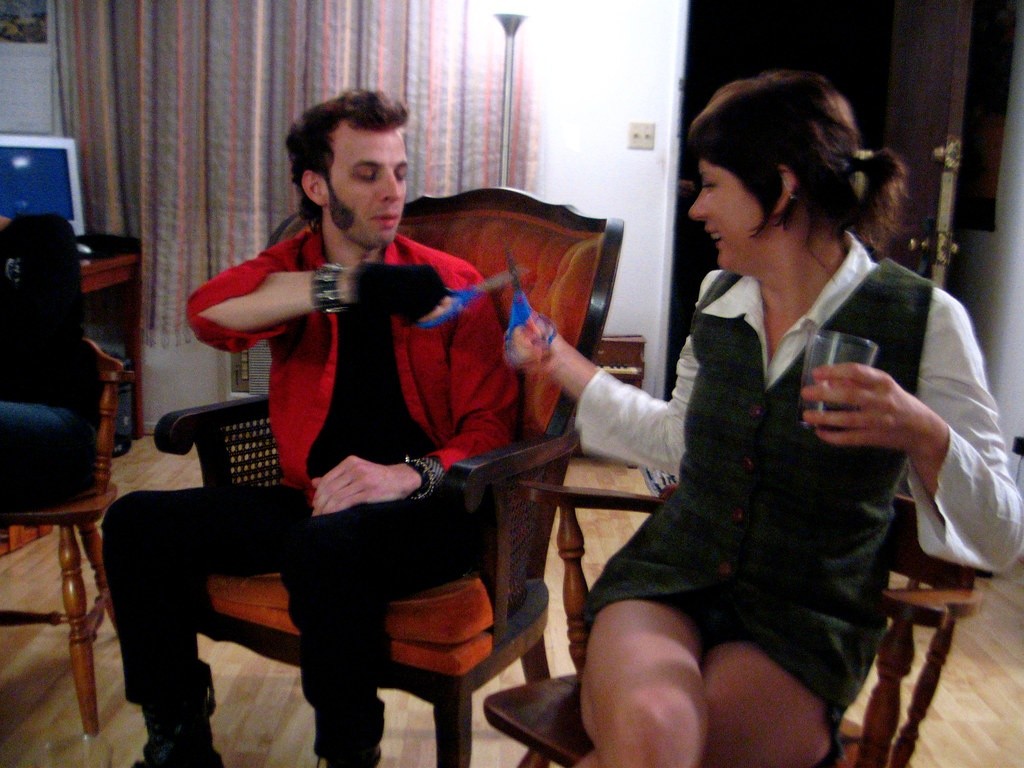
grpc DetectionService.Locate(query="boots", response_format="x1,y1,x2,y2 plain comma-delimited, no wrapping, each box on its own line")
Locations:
132,658,225,768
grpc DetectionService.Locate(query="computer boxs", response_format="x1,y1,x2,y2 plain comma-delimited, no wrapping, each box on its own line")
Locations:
104,351,132,457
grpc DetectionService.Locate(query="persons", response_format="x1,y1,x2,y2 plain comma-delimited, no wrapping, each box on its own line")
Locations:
0,211,99,518
101,86,519,768
505,73,1024,768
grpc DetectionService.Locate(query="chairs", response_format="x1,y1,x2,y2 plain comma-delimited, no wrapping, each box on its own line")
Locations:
0,336,127,738
153,186,624,768
484,480,996,768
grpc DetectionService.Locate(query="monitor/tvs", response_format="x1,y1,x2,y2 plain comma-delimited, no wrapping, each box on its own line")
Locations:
0,133,86,238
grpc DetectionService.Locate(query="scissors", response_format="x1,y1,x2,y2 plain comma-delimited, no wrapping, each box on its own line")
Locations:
407,263,523,331
498,241,560,368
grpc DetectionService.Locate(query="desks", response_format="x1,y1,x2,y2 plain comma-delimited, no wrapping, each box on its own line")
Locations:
80,232,144,441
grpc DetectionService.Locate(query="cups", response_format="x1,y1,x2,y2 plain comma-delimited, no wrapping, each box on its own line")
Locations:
796,330,879,427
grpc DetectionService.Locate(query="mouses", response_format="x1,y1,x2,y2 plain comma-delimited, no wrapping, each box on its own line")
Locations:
76,243,91,253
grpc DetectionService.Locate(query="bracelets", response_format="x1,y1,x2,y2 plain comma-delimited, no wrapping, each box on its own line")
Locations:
313,264,341,310
402,457,444,501
